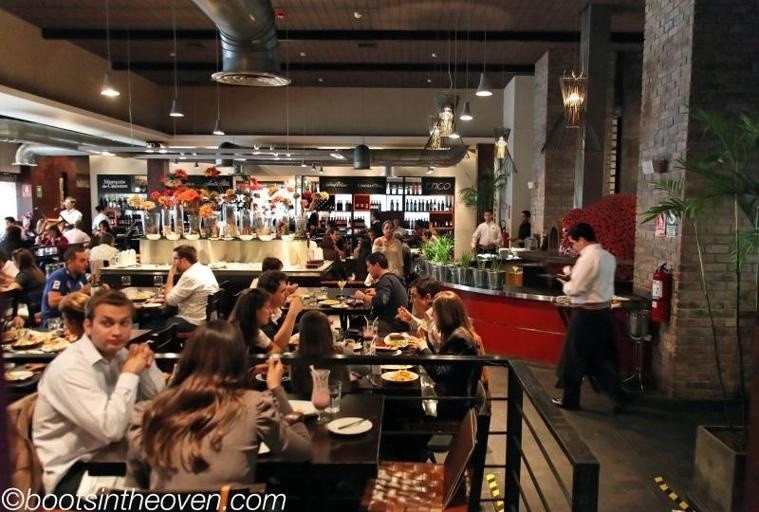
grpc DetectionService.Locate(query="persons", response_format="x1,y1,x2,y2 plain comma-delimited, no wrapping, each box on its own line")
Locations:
507,208,532,247
124,322,313,492
45,196,91,247
249,258,299,299
343,237,372,281
470,210,504,254
32,287,165,512
140,246,221,343
257,271,304,355
394,292,486,461
392,218,406,239
0,212,39,261
403,220,430,246
395,275,443,355
317,228,345,261
354,251,409,331
0,248,46,323
359,221,383,243
89,221,115,248
40,247,111,331
91,200,110,237
227,289,282,355
551,223,631,413
23,184,30,197
306,212,336,236
364,221,403,288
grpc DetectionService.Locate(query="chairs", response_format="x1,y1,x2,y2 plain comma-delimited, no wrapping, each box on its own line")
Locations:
27,289,43,329
387,378,487,465
0,287,21,331
90,245,120,290
217,279,232,324
1,365,41,401
358,407,478,510
149,322,179,374
176,289,225,354
7,390,74,512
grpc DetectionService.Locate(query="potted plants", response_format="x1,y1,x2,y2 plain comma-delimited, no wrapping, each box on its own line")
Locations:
473,246,487,289
432,248,439,279
639,101,758,512
423,240,435,276
437,239,450,283
448,257,458,284
488,250,506,290
457,248,473,286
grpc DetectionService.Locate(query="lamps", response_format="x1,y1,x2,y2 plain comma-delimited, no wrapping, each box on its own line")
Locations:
558,70,587,132
426,165,434,175
329,148,345,160
212,28,225,136
474,2,494,98
458,1,474,121
495,136,508,175
447,42,460,139
168,0,185,118
353,88,370,170
99,1,121,98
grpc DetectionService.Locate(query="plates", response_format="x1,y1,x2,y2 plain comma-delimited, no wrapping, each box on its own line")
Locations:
12,343,41,350
254,374,288,382
318,299,341,304
222,236,236,241
380,371,418,381
2,370,35,381
326,416,373,436
385,331,412,345
287,400,316,416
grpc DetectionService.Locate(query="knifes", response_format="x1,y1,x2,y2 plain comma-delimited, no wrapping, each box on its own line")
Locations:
337,418,368,431
367,376,378,388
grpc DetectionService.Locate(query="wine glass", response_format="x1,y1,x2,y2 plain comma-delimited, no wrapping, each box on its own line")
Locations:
363,321,378,357
121,276,130,288
154,275,163,298
311,370,330,425
337,280,349,300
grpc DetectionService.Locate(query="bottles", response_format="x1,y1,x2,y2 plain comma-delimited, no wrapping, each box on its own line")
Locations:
337,200,352,211
371,200,381,212
405,217,428,229
406,199,451,211
322,216,365,228
390,199,395,211
431,216,452,227
406,183,420,194
396,199,399,211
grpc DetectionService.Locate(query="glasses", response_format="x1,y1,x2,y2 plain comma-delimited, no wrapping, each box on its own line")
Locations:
173,255,182,260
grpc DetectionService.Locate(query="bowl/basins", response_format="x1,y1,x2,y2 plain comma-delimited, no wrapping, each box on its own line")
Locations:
166,232,180,240
238,234,254,242
186,233,201,242
259,235,273,242
146,232,162,242
281,234,294,242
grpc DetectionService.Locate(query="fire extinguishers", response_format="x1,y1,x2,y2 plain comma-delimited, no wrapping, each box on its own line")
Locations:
650,262,673,322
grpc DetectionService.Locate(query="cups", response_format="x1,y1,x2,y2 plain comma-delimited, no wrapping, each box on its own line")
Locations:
323,384,341,414
47,317,60,331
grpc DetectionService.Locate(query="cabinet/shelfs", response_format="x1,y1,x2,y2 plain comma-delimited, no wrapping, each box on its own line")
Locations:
386,175,455,240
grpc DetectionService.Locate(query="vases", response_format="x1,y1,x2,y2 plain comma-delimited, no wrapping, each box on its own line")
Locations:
304,209,317,238
221,204,236,240
160,208,171,237
166,209,184,241
204,217,220,241
275,207,289,236
257,213,276,241
238,210,254,239
183,211,202,240
141,211,160,240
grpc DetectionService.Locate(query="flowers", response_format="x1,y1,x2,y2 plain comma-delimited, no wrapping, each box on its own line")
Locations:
154,189,172,210
161,170,188,193
237,175,257,210
197,187,220,218
269,186,294,209
206,167,234,203
294,187,329,211
127,195,157,213
172,186,200,208
